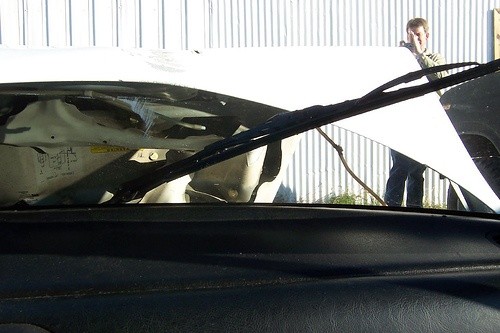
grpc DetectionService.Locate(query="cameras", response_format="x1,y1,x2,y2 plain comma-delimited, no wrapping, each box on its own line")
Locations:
404,43,414,52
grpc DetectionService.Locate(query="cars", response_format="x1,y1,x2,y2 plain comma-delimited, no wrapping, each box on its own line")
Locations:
0,60,500,333
